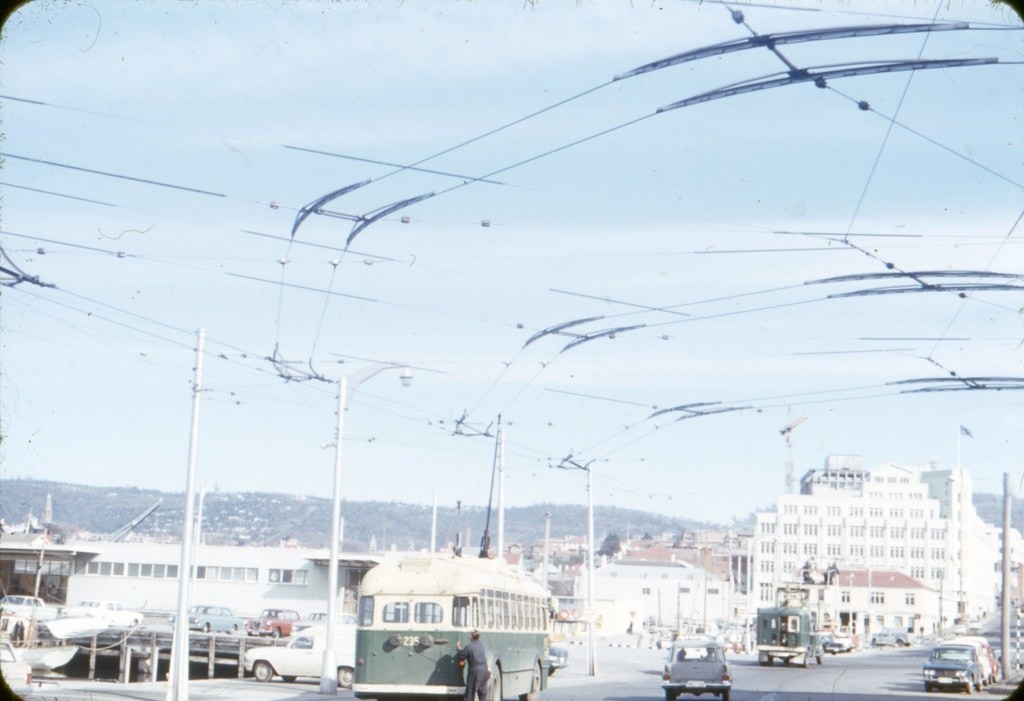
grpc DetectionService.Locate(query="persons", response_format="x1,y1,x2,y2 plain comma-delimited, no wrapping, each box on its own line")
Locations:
456,630,488,701
704,648,715,662
677,650,687,662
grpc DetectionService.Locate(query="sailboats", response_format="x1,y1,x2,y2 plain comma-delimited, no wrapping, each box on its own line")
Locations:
5,530,81,672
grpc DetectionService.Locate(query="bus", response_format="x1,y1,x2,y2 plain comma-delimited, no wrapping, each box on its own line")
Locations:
354,551,557,701
758,607,823,668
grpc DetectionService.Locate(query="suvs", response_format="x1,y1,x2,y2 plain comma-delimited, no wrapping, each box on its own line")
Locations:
924,645,982,694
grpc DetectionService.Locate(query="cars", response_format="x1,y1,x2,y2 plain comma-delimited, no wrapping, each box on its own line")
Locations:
822,631,853,653
1,596,67,621
167,606,244,635
871,627,909,647
248,608,303,638
243,624,355,690
62,601,145,628
662,637,730,700
